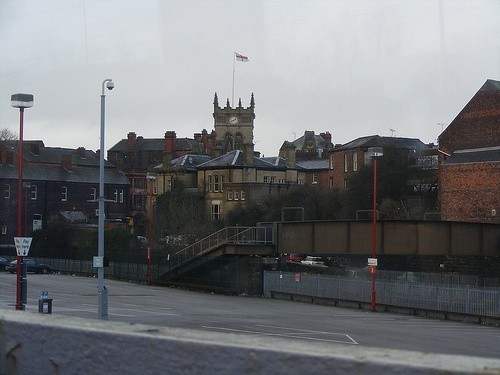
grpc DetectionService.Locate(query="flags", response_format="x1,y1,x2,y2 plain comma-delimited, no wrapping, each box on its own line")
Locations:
235,52,250,63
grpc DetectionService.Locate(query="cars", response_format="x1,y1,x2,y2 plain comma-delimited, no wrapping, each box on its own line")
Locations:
300,255,326,266
0,256,54,274
286,253,305,263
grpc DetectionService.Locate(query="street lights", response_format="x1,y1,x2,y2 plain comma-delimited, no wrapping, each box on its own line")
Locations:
146,176,157,285
368,152,384,311
9,93,34,310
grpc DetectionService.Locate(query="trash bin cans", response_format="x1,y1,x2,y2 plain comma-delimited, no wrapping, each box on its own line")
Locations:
39,298,53,314
